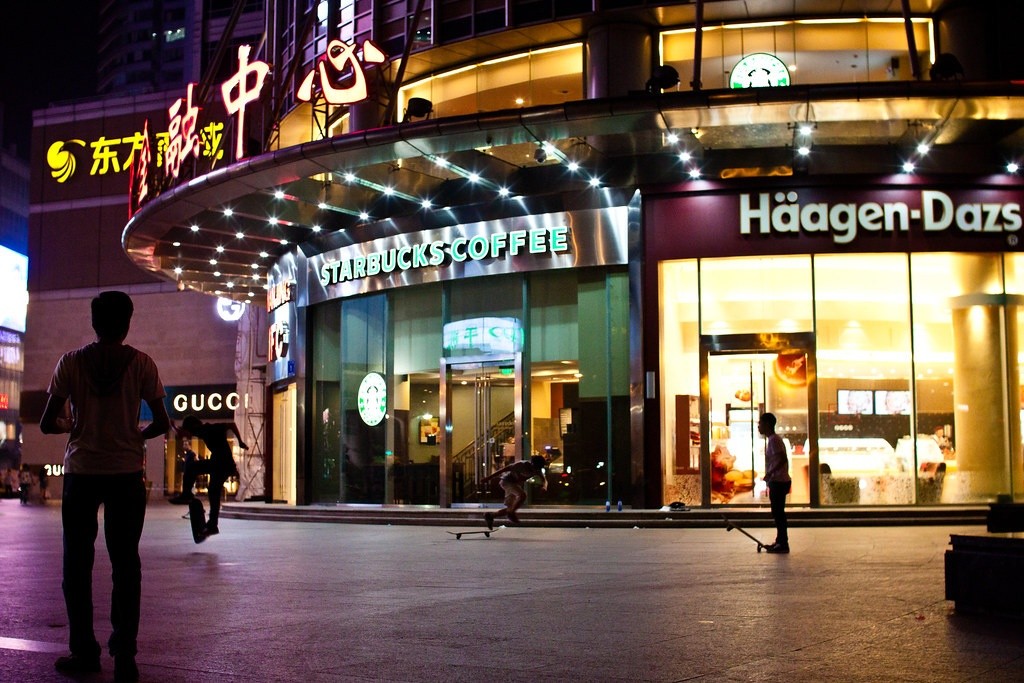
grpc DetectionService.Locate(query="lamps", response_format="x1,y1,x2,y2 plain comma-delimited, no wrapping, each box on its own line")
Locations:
402,98,434,123
646,64,680,93
928,54,967,84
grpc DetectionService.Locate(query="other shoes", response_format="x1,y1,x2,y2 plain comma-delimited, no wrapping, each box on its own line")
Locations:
768,543,789,554
506,512,518,523
197,524,219,538
169,492,195,505
114,658,140,683
484,512,494,529
54,653,101,675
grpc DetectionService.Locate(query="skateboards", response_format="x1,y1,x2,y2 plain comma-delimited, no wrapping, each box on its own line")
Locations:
719,512,773,557
444,528,500,539
188,497,207,545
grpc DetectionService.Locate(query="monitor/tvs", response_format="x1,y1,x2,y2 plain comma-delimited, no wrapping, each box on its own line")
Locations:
874,390,911,415
837,388,874,414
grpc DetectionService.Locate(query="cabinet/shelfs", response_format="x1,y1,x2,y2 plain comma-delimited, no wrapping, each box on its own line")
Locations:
376,463,466,504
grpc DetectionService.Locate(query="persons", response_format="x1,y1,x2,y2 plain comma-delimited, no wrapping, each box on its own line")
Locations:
367,441,440,503
0,462,16,498
171,414,250,538
18,464,49,505
182,439,197,519
496,437,564,477
480,455,548,530
39,291,171,683
758,412,791,553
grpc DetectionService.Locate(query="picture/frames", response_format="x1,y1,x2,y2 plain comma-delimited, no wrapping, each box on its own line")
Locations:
420,417,440,444
559,408,571,437
